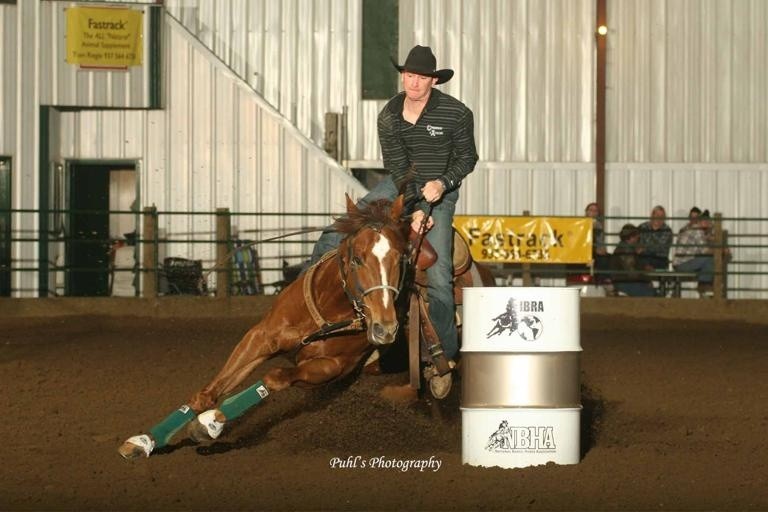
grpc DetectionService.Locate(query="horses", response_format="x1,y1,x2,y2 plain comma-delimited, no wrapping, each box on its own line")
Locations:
115,190,497,463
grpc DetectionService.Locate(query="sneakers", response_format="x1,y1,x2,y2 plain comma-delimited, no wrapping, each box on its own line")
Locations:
429,363,453,400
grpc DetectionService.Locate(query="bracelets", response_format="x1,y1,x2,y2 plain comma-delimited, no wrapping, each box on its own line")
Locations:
436,178,446,191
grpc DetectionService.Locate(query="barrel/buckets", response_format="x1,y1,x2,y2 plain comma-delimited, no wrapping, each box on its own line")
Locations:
460,286,583,470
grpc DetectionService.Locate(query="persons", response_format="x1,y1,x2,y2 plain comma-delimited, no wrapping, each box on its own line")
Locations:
304,44,480,401
609,224,659,298
637,205,671,271
228,225,264,297
577,202,617,301
674,213,715,296
678,205,730,261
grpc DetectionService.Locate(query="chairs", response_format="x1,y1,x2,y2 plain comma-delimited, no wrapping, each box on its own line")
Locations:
164,257,216,296
231,244,284,297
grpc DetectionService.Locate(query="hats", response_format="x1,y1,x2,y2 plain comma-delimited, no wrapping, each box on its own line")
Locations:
388,44,454,85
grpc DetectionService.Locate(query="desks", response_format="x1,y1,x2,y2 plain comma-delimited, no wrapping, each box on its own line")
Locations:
283,260,310,282
645,267,697,298
